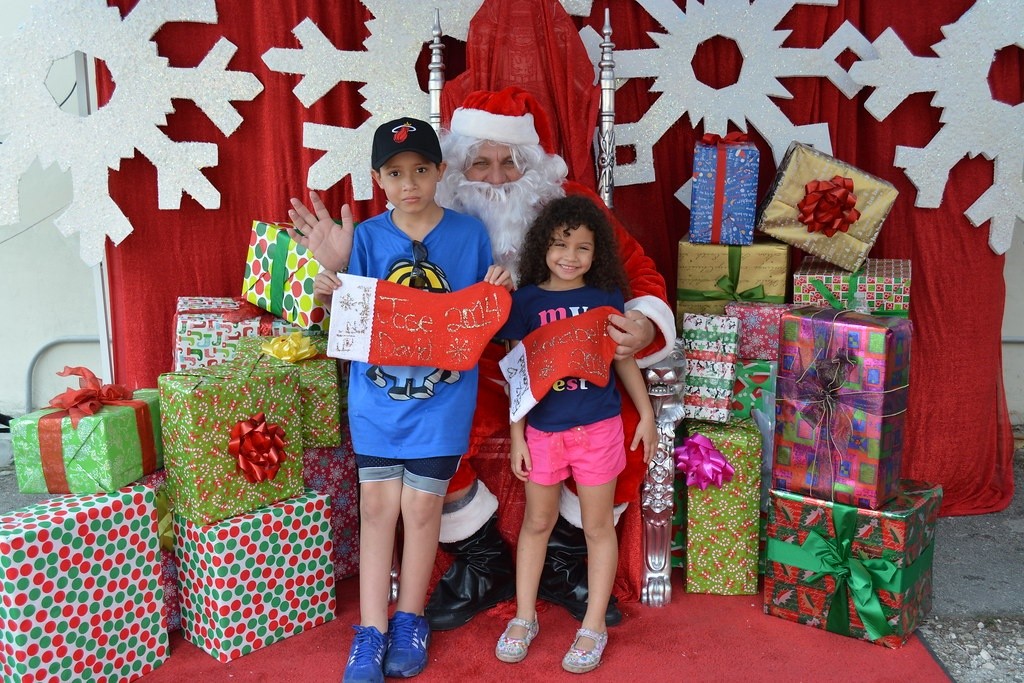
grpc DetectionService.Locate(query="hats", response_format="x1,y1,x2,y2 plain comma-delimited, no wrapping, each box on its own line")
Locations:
372,117,442,170
450,84,568,183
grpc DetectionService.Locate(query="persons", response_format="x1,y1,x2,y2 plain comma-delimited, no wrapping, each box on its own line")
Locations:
313,115,515,683
495,194,660,675
287,82,676,633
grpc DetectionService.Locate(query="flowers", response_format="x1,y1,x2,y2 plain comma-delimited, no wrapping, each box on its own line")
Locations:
50,366,130,426
672,432,736,493
797,174,863,240
791,347,858,466
226,408,288,484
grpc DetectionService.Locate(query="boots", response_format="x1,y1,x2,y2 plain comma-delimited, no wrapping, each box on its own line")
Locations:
423,478,518,631
537,479,629,625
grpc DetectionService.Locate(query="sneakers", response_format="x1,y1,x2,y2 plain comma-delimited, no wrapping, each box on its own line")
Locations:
343,625,389,683
382,610,430,679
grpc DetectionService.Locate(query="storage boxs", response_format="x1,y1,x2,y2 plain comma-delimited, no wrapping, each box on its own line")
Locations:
659,139,941,649
0,216,346,683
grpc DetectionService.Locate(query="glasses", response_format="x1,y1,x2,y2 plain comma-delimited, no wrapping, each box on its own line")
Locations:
409,239,428,290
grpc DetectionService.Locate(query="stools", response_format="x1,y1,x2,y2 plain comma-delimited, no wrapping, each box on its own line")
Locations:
385,9,680,604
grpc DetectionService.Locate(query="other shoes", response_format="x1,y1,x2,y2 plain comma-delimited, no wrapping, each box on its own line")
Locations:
562,629,608,673
496,606,537,661
